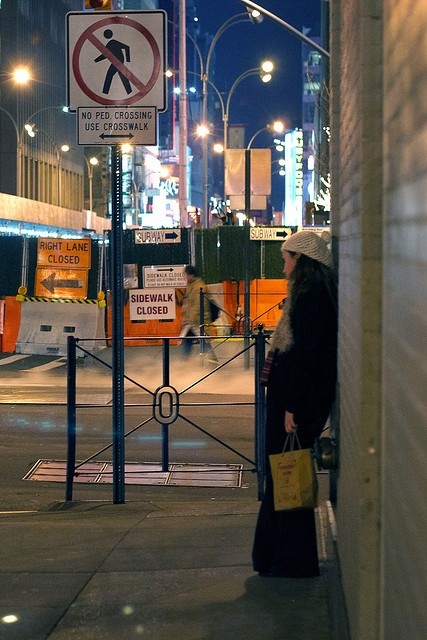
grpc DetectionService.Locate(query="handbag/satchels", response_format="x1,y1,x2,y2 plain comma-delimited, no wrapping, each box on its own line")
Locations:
268,429,317,512
208,299,220,322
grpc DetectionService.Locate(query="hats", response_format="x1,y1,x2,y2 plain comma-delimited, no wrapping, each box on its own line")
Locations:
280,231,333,269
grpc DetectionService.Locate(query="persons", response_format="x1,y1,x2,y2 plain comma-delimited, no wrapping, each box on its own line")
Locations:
175,265,220,369
252,230,338,580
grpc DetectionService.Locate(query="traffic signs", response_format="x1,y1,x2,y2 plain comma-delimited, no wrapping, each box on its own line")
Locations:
143,264,189,289
76,106,158,148
133,228,180,244
248,227,291,242
34,237,91,299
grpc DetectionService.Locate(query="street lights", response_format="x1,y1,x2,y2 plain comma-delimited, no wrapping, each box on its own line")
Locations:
121,166,169,228
273,169,286,176
60,143,132,212
164,60,274,227
271,158,284,165
0,104,69,197
24,123,73,206
165,8,259,227
247,119,284,149
262,145,283,152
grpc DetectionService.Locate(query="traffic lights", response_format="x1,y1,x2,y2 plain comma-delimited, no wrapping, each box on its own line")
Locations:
85,0,113,10
